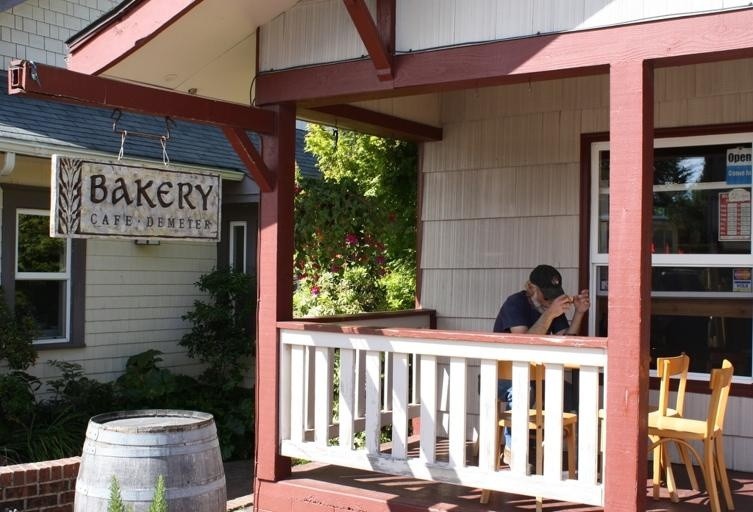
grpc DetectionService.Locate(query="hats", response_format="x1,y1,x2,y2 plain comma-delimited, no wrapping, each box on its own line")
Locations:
530,265,564,299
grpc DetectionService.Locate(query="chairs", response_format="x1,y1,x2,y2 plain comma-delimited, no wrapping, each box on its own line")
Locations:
594,349,736,511
477,353,580,512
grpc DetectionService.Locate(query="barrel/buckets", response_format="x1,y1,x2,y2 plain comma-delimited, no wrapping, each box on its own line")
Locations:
72,407,229,512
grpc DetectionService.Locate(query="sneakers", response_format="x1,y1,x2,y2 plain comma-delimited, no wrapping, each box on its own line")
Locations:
504,449,533,474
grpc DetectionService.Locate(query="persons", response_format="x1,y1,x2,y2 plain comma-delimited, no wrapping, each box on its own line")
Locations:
476,264,590,475
